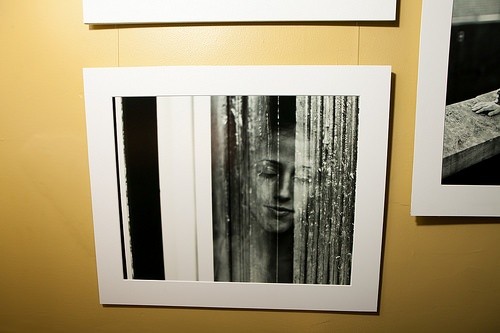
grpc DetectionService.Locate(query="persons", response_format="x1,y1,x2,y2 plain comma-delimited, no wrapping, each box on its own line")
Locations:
471,90,500,117
208,123,317,283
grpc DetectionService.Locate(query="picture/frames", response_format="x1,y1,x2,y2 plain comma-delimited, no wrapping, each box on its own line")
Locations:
82,64,392,314
411,0,499,216
